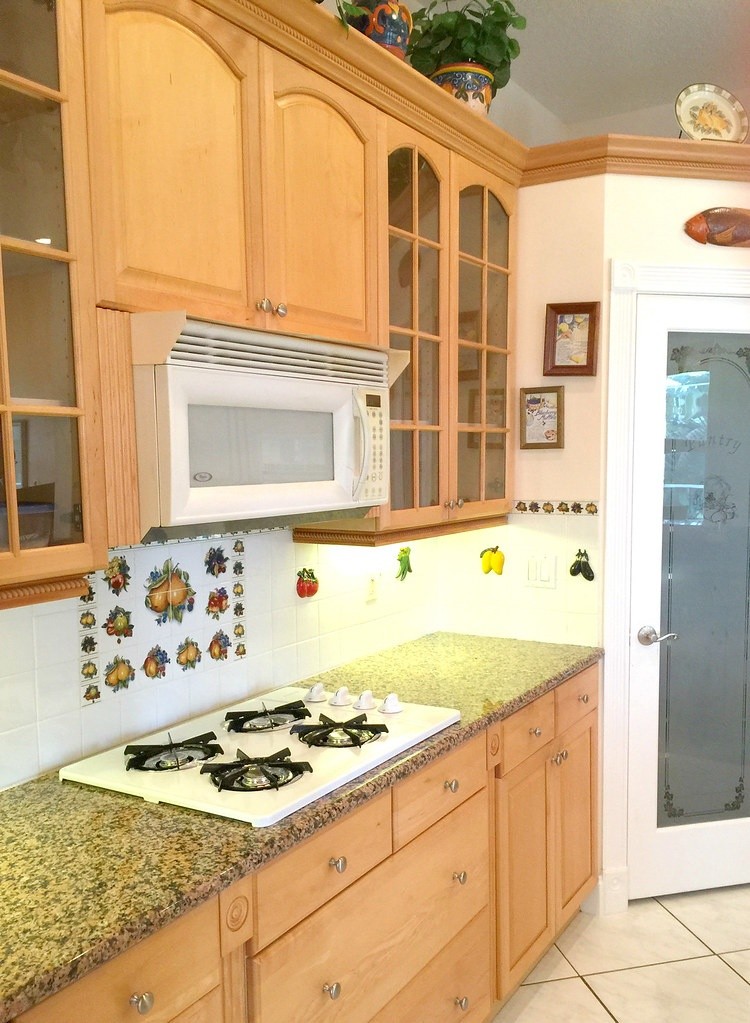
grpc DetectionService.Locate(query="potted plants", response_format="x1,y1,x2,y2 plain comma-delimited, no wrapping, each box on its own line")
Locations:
335,0,527,116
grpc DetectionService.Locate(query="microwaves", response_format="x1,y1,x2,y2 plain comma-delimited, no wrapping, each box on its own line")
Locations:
132,365,390,529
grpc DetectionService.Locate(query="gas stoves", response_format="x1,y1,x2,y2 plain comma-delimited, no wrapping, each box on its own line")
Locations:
59,682,462,826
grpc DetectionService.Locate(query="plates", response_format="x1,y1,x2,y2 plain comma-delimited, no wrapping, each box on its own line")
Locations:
673,82,750,144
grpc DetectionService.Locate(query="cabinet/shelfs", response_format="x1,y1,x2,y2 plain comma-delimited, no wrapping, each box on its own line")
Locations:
0,663,599,1023
0,0,530,610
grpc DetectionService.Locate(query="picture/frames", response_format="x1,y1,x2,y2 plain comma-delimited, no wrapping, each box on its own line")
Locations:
520,386,564,449
468,388,506,449
543,302,600,376
435,310,487,380
